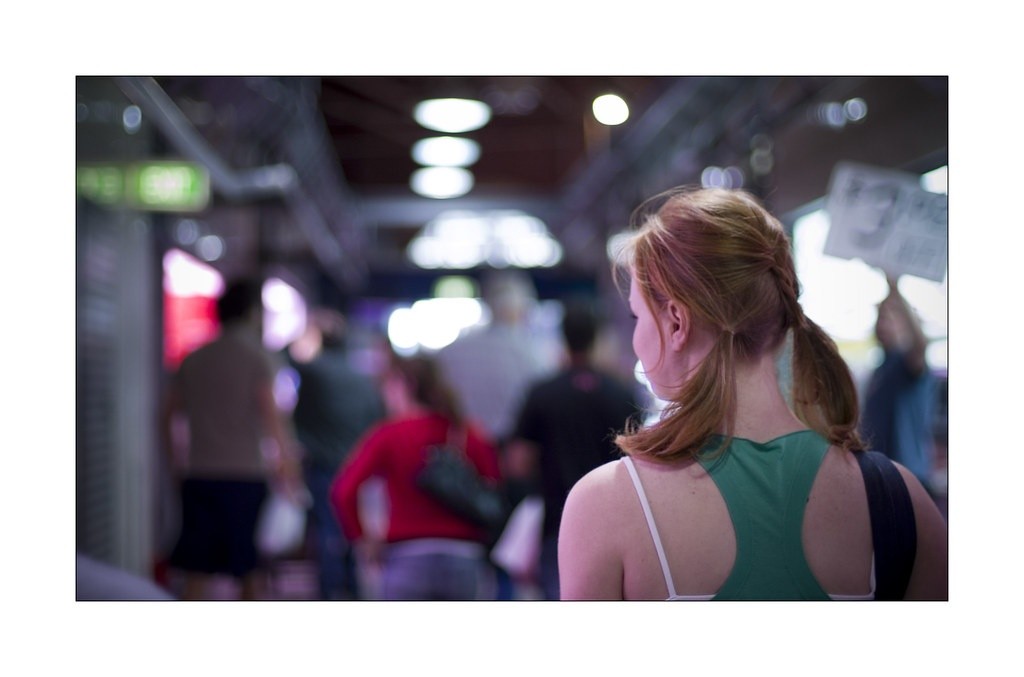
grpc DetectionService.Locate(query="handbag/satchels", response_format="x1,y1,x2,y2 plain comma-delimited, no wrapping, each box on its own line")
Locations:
415,443,511,526
488,494,545,578
255,490,308,555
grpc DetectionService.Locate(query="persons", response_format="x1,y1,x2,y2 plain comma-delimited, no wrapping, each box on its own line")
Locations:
559,189,948,602
862,292,948,514
158,266,639,601
